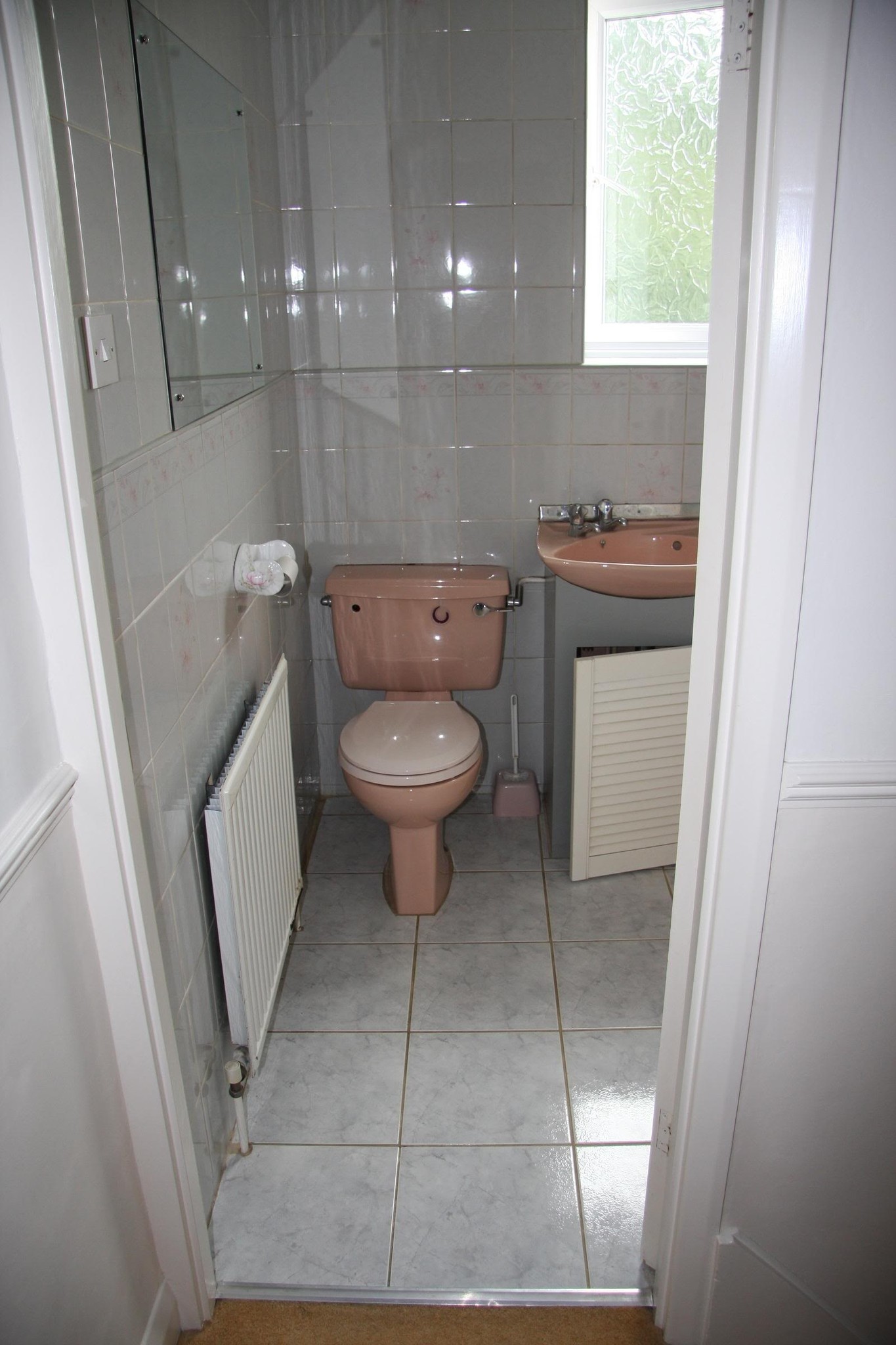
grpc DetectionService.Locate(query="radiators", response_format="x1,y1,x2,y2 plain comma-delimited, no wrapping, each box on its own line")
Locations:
202,650,308,1082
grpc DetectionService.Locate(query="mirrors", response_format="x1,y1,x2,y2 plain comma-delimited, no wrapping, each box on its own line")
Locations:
124,0,268,435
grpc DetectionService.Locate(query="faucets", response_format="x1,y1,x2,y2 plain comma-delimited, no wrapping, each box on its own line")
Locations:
564,503,601,539
597,499,628,531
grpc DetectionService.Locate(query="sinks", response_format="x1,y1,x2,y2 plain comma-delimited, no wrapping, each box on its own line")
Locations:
536,502,701,599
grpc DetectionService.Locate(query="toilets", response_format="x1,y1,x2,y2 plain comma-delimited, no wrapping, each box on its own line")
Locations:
320,562,524,919
